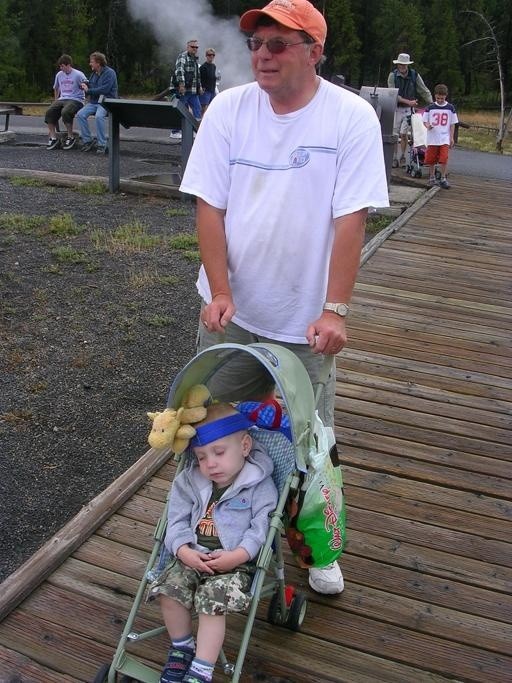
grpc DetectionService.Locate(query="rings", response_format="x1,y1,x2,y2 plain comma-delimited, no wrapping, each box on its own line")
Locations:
203,320,208,328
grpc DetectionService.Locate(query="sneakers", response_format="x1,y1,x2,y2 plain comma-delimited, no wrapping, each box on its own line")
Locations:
307,560,345,595
158,646,205,683
46,134,106,155
170,132,183,138
392,156,407,168
428,179,450,189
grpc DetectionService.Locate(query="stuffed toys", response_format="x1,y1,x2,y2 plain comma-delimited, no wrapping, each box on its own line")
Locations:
146,384,212,456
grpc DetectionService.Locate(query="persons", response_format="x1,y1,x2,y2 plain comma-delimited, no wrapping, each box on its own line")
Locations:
422,84,458,189
75,51,119,154
144,402,279,683
44,53,87,150
176,0,390,598
169,39,203,139
199,48,217,114
387,52,433,169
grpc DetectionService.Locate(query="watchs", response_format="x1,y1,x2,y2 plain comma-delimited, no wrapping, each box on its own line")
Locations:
322,302,349,317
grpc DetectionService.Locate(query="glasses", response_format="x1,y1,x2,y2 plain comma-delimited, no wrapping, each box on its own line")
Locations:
246,38,306,54
191,46,199,48
208,54,215,56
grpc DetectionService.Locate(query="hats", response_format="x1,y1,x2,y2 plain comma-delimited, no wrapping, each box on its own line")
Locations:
393,53,413,65
240,0,327,48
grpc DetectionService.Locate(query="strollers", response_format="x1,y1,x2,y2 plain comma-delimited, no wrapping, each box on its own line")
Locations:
93,312,336,682
404,98,441,179
